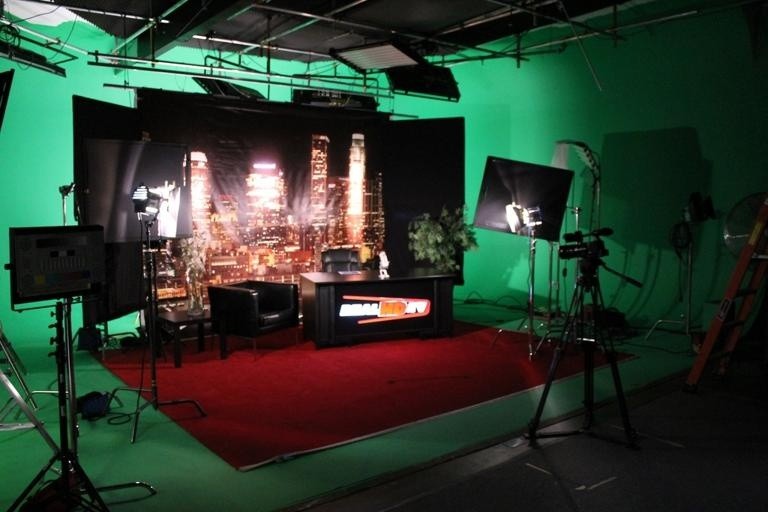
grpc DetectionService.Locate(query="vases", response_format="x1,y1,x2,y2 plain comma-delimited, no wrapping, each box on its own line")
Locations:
186,270,207,316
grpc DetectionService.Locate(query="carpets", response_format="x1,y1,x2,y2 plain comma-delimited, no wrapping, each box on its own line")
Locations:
91,320,639,472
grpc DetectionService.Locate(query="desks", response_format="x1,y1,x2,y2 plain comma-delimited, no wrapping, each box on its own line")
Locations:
300,267,464,346
158,309,227,368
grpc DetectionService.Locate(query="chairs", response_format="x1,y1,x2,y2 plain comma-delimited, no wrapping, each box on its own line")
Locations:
320,248,361,272
208,279,299,359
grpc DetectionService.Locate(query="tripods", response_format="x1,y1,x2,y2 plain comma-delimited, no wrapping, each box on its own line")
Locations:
6,305,112,510
119,251,206,443
527,266,643,453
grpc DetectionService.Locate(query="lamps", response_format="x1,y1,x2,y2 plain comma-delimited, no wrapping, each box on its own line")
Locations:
489,202,543,363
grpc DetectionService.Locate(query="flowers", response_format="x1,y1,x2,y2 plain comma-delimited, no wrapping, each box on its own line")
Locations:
180,229,211,309
